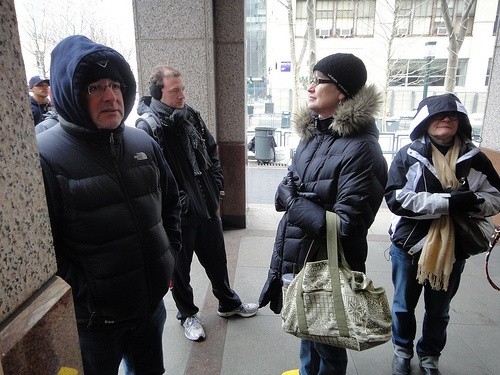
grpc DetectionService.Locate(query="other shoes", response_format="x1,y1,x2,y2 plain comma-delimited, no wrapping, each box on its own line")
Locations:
281,369,300,375
392,353,411,375
419,360,441,375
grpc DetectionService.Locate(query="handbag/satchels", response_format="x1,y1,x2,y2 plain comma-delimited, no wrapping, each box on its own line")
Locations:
281,210,393,352
443,178,496,260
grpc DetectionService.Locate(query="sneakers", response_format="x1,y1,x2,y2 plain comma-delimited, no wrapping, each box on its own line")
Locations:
218,302,258,317
181,314,206,341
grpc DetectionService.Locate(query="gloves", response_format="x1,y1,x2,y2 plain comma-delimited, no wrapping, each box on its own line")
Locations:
451,187,486,213
278,170,305,211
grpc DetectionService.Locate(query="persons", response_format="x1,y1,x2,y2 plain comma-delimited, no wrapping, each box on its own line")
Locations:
25,35,262,375
258,52,390,374
382,94,500,375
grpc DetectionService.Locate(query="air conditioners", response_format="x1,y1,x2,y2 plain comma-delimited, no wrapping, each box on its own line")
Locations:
340,28,351,38
397,28,408,36
437,27,447,35
319,29,330,38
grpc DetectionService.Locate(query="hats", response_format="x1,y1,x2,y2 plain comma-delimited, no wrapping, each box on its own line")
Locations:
28,76,50,91
313,53,367,99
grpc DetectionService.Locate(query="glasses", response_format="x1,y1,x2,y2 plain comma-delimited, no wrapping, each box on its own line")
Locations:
433,112,460,122
81,80,121,96
309,77,336,86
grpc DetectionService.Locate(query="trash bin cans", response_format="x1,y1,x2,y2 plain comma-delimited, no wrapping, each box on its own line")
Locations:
247,106,254,114
265,103,274,113
281,111,291,128
255,127,276,165
